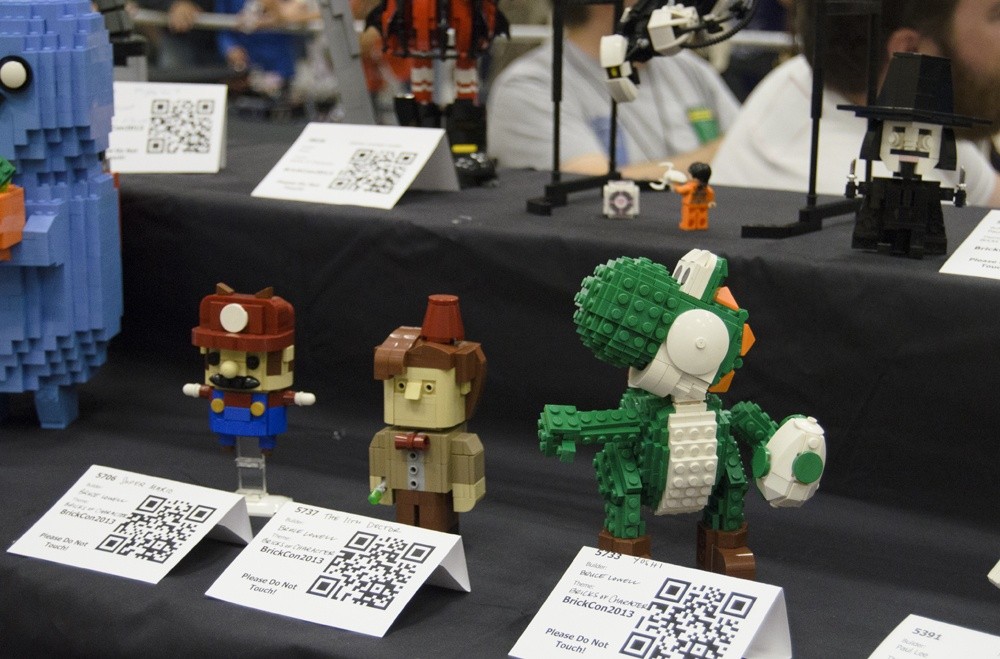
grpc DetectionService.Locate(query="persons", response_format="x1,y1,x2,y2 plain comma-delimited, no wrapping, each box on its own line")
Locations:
483,0,743,187
158,0,340,126
708,0,1000,209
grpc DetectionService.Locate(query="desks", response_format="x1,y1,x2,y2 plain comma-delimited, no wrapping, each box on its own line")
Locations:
0,120,1000,659
